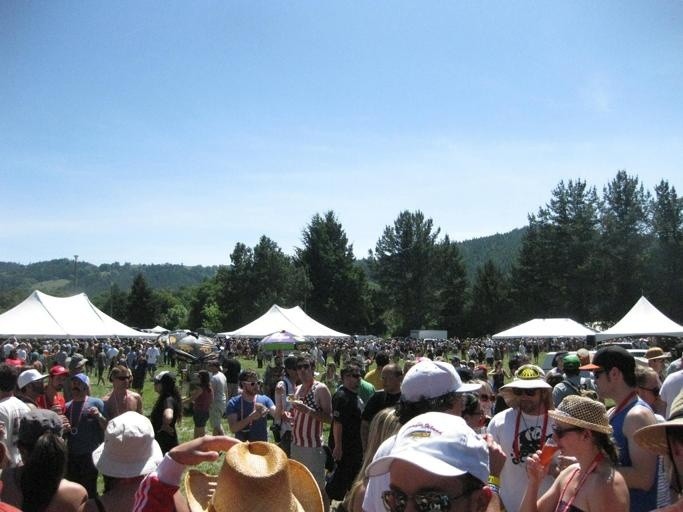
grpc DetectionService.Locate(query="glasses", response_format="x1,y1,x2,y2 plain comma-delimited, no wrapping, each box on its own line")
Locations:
594,369,607,379
551,422,579,438
480,394,496,402
639,386,659,397
381,489,474,511
512,388,538,396
243,380,261,386
297,364,312,370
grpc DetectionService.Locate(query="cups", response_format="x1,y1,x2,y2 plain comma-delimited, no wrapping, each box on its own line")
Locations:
538,438,558,465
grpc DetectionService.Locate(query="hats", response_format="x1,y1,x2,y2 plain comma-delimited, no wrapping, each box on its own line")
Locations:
400,359,484,401
13,409,64,442
90,410,165,478
1,339,222,391
547,393,614,434
645,347,672,361
563,355,579,370
364,412,492,482
579,346,636,373
633,387,683,458
183,439,325,512
497,363,552,390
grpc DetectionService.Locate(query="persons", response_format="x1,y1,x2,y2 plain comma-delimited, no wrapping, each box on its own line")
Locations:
0,329,682,511
